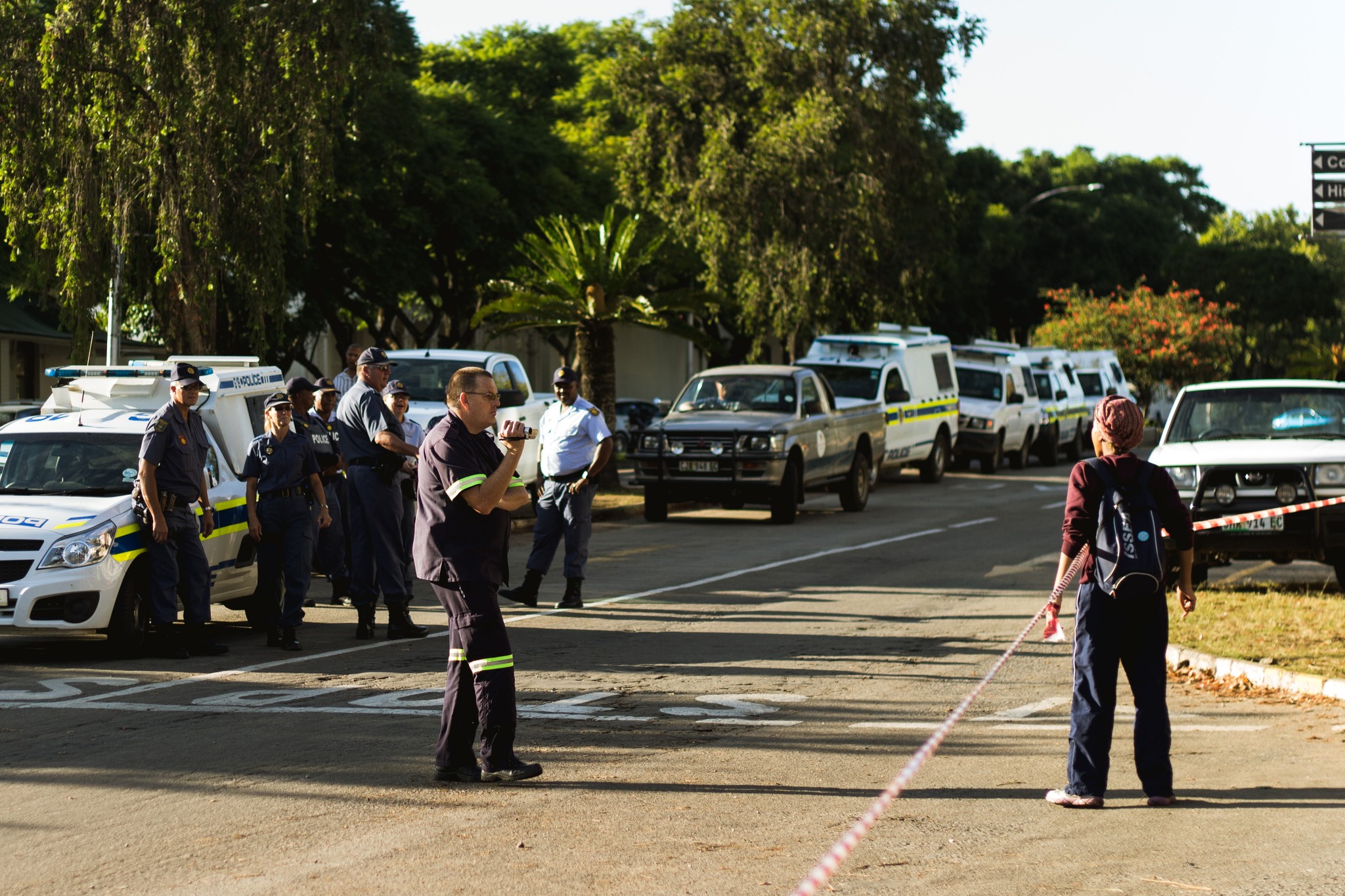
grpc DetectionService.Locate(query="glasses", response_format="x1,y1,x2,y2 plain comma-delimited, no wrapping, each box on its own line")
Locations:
391,395,410,400
184,385,200,390
269,406,292,411
367,366,390,371
457,392,501,400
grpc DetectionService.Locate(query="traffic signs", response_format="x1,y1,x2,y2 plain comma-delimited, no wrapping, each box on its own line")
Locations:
1312,151,1345,230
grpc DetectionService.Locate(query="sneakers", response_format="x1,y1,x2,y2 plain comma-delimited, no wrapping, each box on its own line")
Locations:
1147,793,1176,807
1045,788,1103,807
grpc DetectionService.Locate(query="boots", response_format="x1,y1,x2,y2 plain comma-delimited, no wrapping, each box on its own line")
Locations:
153,623,188,659
555,578,584,609
498,570,543,606
186,621,230,655
386,606,429,638
357,604,376,639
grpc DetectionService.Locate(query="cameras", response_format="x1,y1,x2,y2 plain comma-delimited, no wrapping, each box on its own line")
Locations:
522,427,538,440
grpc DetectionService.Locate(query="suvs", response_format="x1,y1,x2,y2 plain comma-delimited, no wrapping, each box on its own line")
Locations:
1148,379,1345,592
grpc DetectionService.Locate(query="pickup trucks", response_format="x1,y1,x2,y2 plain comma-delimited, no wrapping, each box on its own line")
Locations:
751,324,962,492
951,339,1138,473
384,349,559,517
628,365,886,524
0,355,296,661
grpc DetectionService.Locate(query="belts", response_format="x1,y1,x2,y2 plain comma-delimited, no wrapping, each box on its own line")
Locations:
545,468,588,482
261,485,306,498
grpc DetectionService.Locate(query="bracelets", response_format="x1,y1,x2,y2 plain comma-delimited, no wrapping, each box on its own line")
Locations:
496,500,501,507
202,506,211,511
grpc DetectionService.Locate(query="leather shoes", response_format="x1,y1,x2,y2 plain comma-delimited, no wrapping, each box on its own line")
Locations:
432,764,482,782
481,758,542,782
284,626,301,651
269,625,283,646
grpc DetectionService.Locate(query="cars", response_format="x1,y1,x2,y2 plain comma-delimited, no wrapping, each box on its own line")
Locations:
1272,407,1332,430
613,397,658,460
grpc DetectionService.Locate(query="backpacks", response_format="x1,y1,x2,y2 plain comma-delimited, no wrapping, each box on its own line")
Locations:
1082,458,1169,597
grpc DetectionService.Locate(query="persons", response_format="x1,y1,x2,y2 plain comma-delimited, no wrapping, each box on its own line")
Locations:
412,366,543,782
678,378,752,412
332,344,365,410
334,347,429,641
498,366,614,608
242,392,333,651
1046,393,1196,808
284,376,425,607
131,362,231,658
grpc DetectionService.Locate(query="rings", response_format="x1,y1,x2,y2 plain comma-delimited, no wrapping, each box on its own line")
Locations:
573,484,576,487
328,523,330,524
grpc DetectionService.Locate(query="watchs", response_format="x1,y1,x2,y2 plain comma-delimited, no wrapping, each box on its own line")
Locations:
321,504,328,509
582,471,592,480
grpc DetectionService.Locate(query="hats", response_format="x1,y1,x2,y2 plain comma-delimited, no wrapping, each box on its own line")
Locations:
264,393,294,411
285,377,322,395
383,380,411,396
314,378,342,394
551,367,578,386
357,347,398,367
171,362,204,387
1096,396,1145,450
